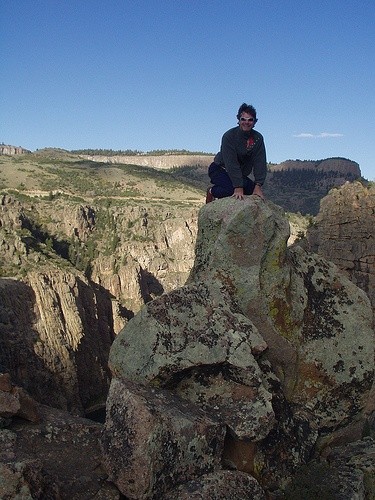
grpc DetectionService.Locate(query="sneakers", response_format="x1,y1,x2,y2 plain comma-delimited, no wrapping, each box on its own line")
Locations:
206,187,215,204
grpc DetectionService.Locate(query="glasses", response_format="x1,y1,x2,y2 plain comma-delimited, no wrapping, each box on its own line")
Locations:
239,117,255,122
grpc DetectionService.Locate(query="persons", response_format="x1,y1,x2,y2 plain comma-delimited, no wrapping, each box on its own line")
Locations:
205,103,266,205
